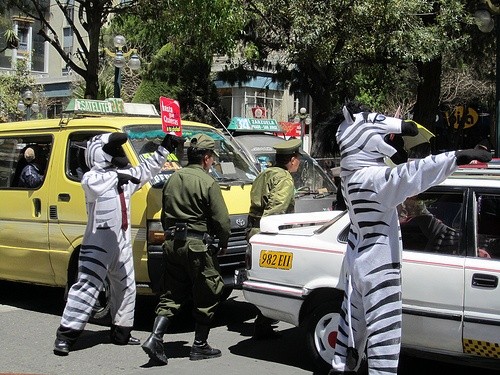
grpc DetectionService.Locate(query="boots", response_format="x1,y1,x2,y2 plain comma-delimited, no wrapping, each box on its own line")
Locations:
189,322,222,361
141,316,168,365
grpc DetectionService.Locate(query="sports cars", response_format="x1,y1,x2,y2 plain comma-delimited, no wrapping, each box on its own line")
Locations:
242,156,499,370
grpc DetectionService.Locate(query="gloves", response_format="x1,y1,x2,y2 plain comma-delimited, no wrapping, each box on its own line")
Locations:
455,144,492,166
118,172,140,185
160,134,181,153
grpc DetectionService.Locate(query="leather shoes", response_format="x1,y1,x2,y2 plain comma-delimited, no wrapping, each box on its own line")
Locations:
112,325,140,344
54,338,69,353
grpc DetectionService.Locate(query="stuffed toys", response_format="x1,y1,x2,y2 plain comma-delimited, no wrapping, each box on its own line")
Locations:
329,101,493,375
53,132,187,354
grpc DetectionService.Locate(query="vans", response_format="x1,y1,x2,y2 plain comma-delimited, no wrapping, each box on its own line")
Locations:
224,116,338,212
0,98,264,321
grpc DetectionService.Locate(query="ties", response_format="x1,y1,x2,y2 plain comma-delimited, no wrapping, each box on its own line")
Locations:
117,187,129,231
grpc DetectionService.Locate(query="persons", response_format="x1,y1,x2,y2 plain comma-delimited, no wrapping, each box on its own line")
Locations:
141,133,232,366
245,139,302,326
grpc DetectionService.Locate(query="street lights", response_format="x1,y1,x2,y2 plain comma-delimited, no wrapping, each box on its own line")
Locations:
100,34,141,96
293,107,312,151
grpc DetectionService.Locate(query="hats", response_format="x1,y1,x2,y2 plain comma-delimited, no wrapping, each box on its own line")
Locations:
190,134,220,158
272,139,304,157
